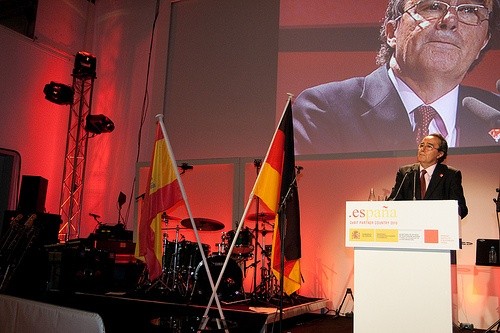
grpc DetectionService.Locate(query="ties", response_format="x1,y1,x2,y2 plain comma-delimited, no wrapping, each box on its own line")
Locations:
420,170,428,200
414,106,437,143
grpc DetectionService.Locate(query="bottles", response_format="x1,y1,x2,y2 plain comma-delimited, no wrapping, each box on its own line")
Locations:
367,188,376,201
489,246,496,263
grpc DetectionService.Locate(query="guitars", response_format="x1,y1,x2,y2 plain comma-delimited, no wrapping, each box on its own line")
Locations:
0,211,38,294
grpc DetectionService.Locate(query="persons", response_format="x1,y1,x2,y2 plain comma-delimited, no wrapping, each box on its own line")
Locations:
292,0,500,156
387,133,468,220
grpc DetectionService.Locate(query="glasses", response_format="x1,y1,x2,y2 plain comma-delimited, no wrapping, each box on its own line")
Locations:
395,0,489,26
418,144,438,151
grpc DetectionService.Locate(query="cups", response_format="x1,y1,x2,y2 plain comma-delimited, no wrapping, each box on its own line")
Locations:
378,196,388,201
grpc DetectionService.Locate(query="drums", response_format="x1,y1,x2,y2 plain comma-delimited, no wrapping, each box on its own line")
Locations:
163,240,210,274
226,229,254,256
194,255,243,299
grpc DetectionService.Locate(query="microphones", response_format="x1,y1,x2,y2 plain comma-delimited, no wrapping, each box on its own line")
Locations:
89,213,100,218
178,165,193,169
462,97,500,129
413,166,418,200
295,165,303,170
392,169,411,200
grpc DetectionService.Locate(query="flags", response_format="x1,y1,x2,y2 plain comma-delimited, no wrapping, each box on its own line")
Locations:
135,121,183,280
253,98,302,296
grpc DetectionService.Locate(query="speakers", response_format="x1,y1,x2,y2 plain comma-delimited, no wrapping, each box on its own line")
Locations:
17,175,48,213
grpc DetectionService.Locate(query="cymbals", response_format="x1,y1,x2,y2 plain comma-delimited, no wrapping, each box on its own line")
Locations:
247,213,276,221
181,217,224,232
161,215,182,221
